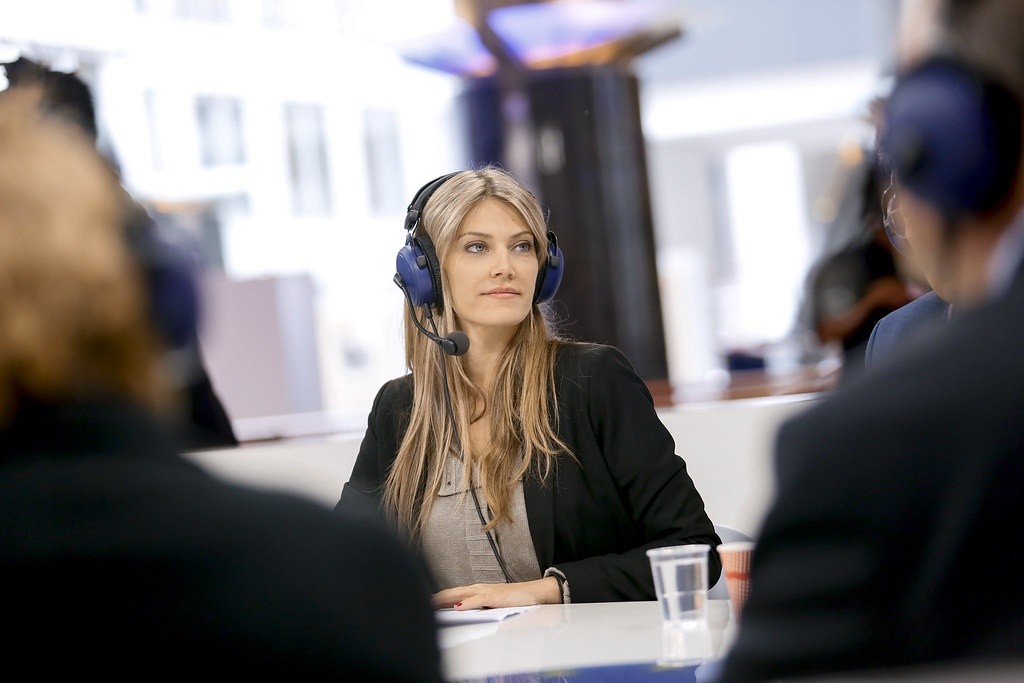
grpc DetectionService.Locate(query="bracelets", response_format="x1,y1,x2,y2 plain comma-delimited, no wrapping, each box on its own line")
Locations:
549,572,564,603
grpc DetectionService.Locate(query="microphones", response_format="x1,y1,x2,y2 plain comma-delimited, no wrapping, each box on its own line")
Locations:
402,287,470,355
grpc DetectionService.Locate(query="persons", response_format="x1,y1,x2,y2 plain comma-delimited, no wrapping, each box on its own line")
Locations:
334,168,723,610
0,58,447,682
719,0,1024,683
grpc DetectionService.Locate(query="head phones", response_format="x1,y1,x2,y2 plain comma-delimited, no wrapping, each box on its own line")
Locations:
884,0,1024,238
394,170,566,304
115,203,201,351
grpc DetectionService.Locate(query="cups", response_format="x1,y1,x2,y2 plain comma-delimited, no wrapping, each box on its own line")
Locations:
716,542,754,627
654,621,716,669
645,544,712,622
